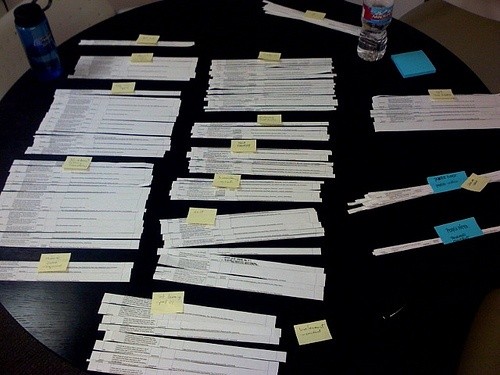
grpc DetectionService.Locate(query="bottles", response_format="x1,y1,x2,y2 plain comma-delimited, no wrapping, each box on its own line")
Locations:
356,0,394,61
13,2,61,82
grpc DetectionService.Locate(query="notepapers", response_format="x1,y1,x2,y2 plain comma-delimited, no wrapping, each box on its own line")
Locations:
391,50,437,79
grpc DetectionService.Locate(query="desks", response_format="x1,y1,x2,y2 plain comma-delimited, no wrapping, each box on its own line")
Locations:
0,0,500,375
